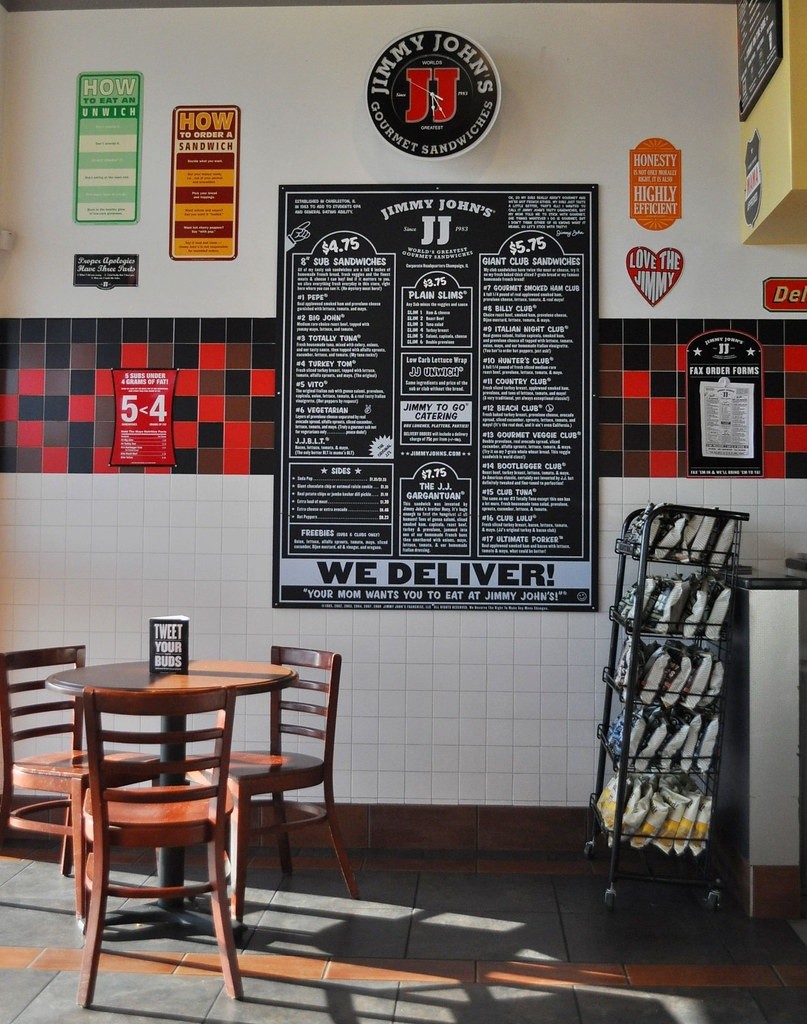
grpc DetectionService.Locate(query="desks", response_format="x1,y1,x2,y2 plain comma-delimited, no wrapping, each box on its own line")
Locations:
45,660,301,942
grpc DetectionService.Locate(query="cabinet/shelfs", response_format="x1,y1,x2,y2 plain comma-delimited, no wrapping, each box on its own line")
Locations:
582,502,750,914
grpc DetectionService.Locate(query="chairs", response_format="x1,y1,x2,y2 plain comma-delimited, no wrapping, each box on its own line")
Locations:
78,685,243,1010
0,645,159,922
187,645,361,923
